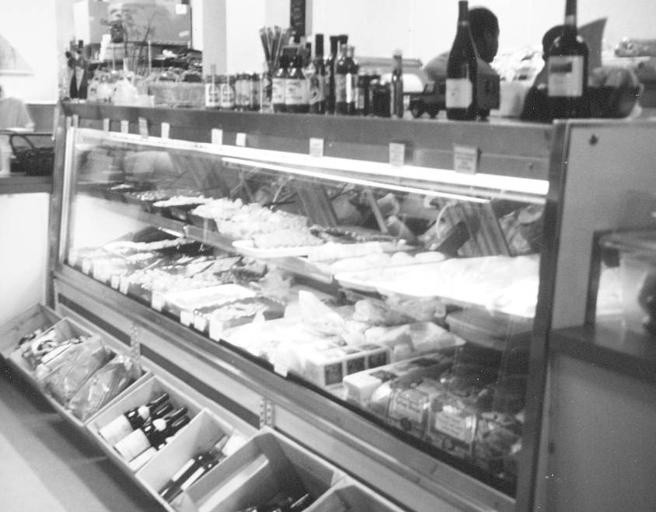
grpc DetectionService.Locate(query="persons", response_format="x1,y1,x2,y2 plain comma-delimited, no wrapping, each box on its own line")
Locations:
425,7,500,110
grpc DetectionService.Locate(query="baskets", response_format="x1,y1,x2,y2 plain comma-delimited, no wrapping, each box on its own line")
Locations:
8,133,54,177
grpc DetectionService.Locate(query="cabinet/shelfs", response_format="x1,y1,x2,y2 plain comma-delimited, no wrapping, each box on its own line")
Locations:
0,81,656,512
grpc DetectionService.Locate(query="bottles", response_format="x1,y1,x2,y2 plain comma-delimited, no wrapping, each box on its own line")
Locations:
305,43,315,87
166,416,189,436
204,75,220,111
347,46,357,115
250,72,260,112
135,403,173,428
96,392,169,442
310,34,326,116
243,497,293,512
163,453,224,503
235,74,250,111
325,35,338,116
339,36,348,45
333,44,348,116
158,437,227,497
354,68,366,117
388,53,405,117
547,0,589,123
286,493,310,512
286,55,309,113
220,75,235,111
112,407,187,463
271,55,290,113
443,0,478,121
127,435,173,474
